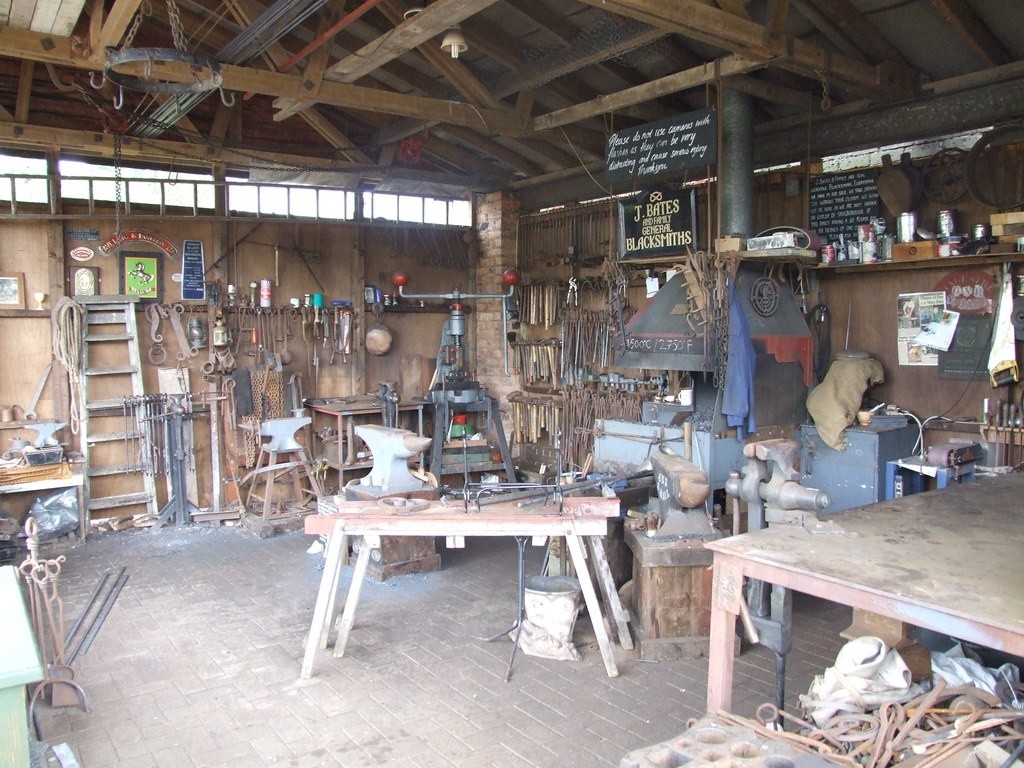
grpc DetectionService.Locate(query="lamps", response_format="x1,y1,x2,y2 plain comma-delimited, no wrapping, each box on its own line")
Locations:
440,29,469,60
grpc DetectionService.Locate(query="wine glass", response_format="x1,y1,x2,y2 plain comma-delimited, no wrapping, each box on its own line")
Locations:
35,293,46,310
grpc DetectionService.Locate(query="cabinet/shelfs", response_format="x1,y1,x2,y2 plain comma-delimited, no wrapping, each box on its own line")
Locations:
0,563,54,768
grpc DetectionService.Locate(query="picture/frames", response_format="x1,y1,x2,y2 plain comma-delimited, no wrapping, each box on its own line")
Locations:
0,272,27,311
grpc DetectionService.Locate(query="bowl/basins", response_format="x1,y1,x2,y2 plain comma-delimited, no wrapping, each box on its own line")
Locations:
857,411,875,425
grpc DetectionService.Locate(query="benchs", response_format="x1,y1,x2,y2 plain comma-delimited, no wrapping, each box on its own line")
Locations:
299,511,621,678
318,493,634,659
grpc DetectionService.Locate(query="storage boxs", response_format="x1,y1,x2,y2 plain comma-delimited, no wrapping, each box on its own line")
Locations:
891,240,942,261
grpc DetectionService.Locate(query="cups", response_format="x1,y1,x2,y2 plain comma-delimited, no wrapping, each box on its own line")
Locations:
848,242,861,259
881,237,895,261
896,211,918,243
860,242,876,263
835,245,848,260
821,246,835,263
312,294,324,308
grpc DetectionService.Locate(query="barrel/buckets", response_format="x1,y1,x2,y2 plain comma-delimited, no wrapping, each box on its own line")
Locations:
524,575,581,642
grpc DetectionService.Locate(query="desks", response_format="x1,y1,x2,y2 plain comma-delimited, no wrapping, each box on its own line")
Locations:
702,471,1024,733
0,472,87,548
304,396,434,494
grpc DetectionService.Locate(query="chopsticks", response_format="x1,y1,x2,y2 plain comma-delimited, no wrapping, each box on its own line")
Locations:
870,403,885,412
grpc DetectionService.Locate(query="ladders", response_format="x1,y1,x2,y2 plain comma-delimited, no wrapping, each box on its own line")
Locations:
72,294,161,541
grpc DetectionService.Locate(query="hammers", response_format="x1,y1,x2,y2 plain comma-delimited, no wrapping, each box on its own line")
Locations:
510,337,561,387
515,279,559,330
508,391,562,449
